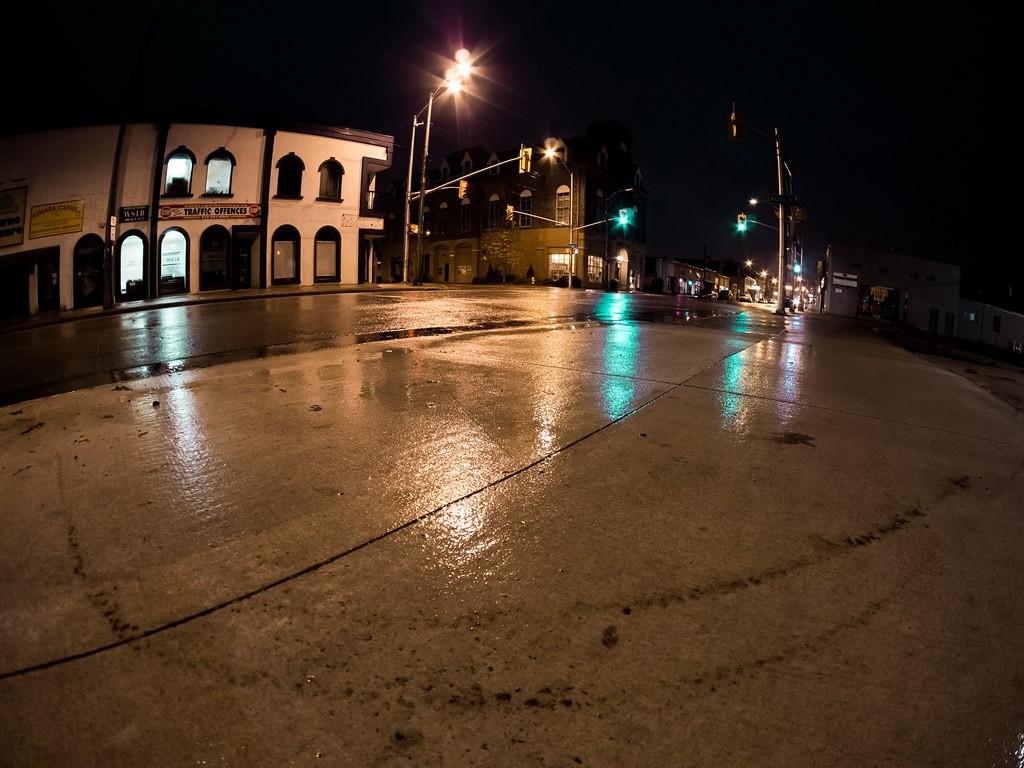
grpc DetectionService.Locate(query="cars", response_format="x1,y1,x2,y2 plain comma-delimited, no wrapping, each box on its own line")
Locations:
769,298,777,304
758,298,768,304
739,293,752,303
699,288,718,301
717,290,733,302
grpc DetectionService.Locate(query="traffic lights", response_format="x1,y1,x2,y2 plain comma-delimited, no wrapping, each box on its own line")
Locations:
793,264,801,274
620,209,627,224
458,180,469,199
506,204,514,222
738,214,746,231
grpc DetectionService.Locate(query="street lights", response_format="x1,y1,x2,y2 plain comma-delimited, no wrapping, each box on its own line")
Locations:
749,198,788,317
402,59,471,284
605,188,632,292
544,149,574,289
